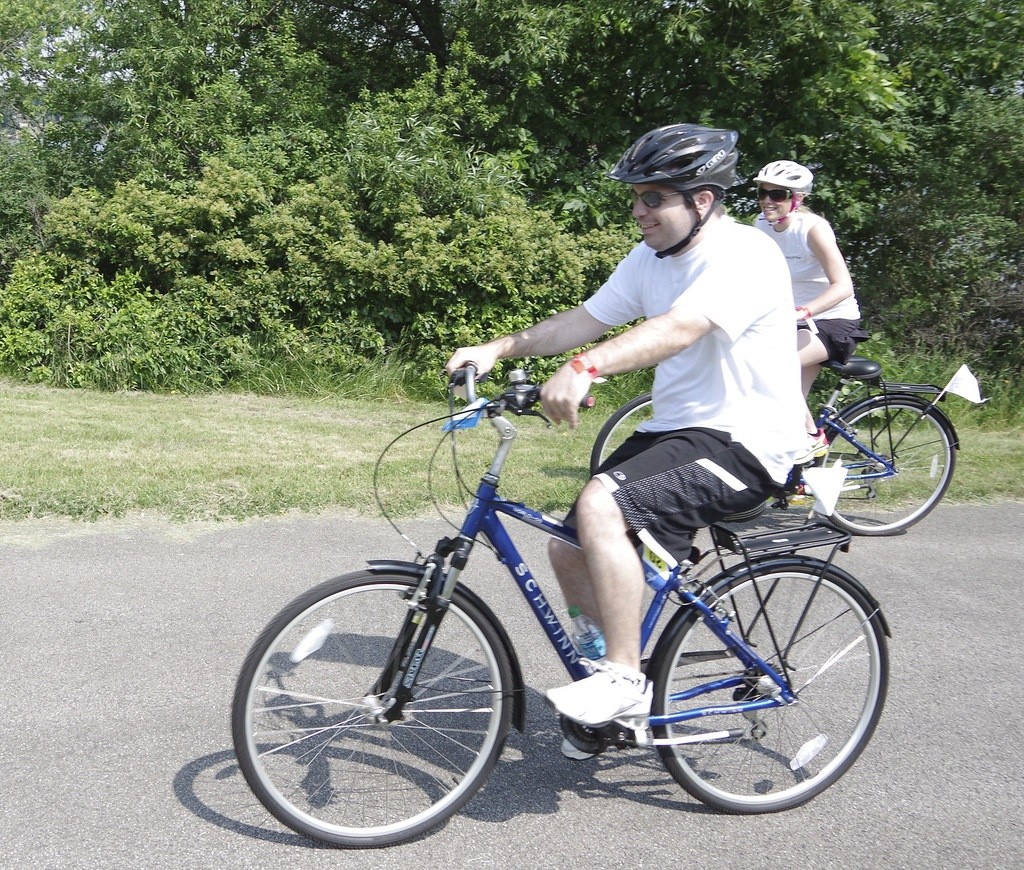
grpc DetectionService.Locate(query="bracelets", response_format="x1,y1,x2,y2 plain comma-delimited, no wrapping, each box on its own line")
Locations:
571,352,608,383
795,305,819,335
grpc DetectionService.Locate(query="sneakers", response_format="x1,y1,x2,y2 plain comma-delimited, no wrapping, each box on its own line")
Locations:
794,427,830,466
544,656,654,732
560,738,596,760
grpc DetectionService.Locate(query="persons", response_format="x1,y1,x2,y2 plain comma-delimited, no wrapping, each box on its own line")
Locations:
445,123,798,761
752,160,861,498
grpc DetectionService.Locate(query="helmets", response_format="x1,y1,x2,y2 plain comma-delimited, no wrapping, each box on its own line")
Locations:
606,123,739,202
753,159,814,213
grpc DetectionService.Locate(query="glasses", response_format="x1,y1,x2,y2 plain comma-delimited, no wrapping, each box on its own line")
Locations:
757,185,794,204
629,187,700,208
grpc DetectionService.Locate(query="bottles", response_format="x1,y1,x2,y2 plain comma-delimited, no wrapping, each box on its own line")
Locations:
569,609,608,672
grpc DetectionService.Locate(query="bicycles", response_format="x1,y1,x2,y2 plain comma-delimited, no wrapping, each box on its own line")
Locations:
587,351,961,537
231,361,892,852
